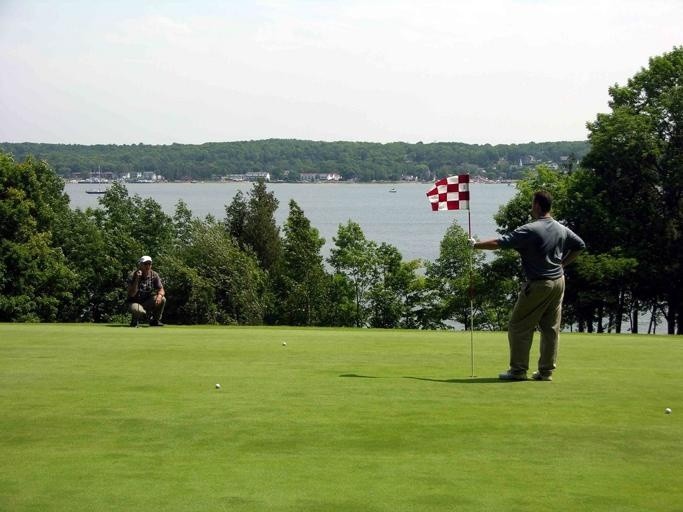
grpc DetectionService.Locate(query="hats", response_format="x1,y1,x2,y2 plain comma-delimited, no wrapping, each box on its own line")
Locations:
140,256,152,263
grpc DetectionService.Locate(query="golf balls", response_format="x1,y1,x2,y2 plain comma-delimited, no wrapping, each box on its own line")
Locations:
665,408,672,413
283,343,286,346
216,384,220,388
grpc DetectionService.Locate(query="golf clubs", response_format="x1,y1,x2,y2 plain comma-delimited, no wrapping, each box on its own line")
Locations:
137,272,140,328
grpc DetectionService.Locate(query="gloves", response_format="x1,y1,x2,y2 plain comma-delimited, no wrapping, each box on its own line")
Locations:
467,236,476,249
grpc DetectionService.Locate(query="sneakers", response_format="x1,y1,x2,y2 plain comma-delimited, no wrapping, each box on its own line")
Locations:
150,319,163,326
500,370,527,380
532,371,552,381
132,316,137,326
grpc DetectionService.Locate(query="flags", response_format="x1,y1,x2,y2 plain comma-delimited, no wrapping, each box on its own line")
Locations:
427,174,469,211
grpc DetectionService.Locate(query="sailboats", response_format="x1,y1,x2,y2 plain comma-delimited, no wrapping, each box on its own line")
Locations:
86,166,107,194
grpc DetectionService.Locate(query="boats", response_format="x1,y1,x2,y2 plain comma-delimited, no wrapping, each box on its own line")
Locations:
388,188,396,193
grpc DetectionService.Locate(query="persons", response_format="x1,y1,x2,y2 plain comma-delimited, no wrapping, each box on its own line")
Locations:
127,256,168,327
466,190,587,382
